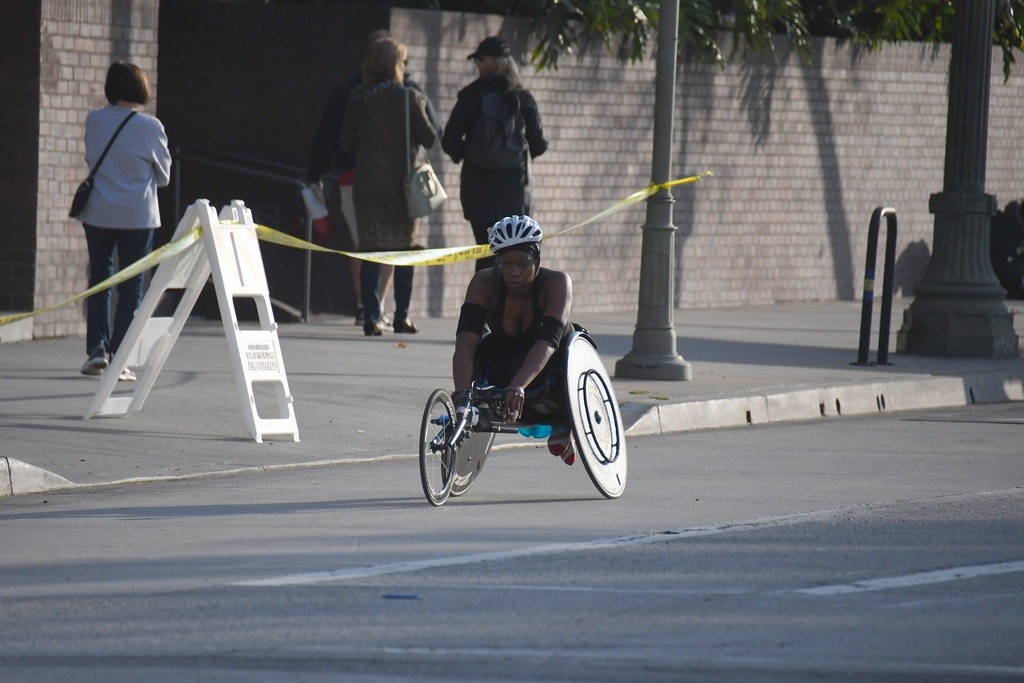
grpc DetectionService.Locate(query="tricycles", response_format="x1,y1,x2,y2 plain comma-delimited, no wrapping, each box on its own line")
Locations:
420,323,629,508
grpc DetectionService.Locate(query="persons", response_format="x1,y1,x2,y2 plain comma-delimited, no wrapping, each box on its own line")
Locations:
449,214,573,424
338,37,438,337
442,36,550,269
80,60,174,379
303,27,441,328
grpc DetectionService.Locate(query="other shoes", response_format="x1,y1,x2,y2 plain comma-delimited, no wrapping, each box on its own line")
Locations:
81,349,107,376
363,320,382,335
118,368,137,382
393,317,417,333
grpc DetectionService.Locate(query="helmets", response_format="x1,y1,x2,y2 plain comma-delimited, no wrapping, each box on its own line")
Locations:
488,215,542,253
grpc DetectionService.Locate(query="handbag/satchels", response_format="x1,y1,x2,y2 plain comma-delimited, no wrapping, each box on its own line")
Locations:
68,176,94,218
404,164,448,220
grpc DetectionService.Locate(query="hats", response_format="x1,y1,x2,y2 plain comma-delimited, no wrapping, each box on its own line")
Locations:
467,36,511,60
